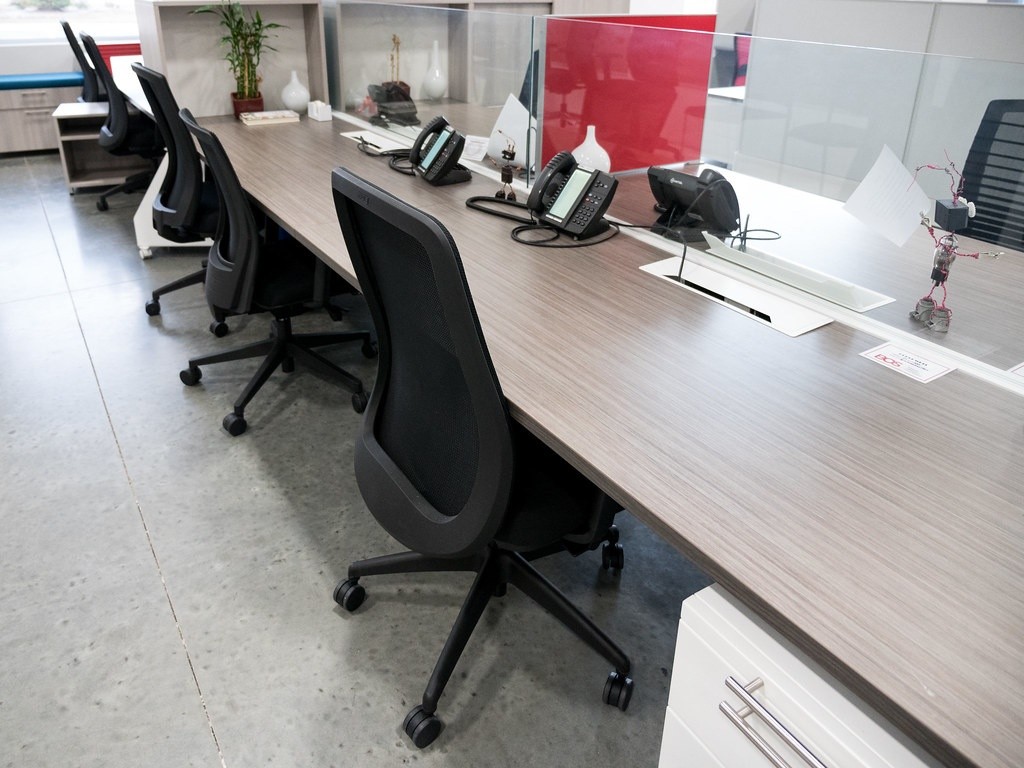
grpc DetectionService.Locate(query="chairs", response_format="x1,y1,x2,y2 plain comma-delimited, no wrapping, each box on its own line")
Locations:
79,32,169,210
953,99,1024,253
332,166,633,751
132,63,342,339
180,106,379,435
59,21,108,103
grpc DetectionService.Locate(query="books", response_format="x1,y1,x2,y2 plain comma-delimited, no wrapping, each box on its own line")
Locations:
239,110,300,125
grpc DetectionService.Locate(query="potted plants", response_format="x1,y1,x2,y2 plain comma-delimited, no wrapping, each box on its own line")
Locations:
190,0,291,121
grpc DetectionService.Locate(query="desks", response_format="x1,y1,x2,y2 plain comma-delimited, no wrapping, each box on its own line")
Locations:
186,95,1024,768
108,53,155,121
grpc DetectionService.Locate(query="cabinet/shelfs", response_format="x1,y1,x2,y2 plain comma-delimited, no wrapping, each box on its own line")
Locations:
0,72,86,154
133,0,632,116
52,101,147,194
657,582,946,767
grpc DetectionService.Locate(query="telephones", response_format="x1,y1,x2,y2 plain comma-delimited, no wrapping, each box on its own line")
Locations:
646,163,741,235
526,150,619,240
409,114,473,186
